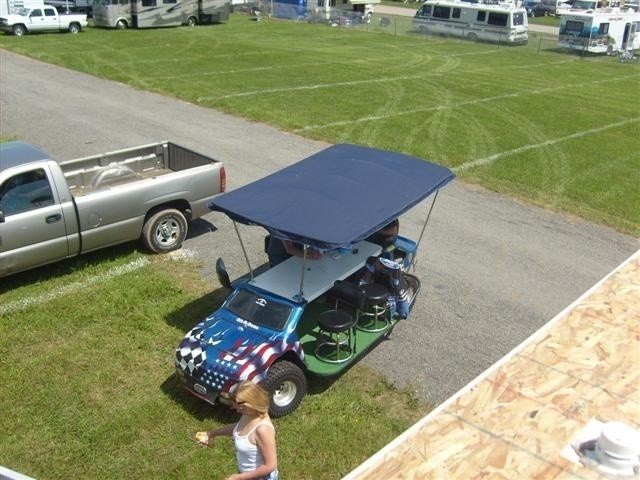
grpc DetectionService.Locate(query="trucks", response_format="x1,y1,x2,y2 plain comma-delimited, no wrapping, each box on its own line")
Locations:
92,0,230,29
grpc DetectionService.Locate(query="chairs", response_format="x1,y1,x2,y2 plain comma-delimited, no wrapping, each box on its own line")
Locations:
216,234,407,363
607,44,640,63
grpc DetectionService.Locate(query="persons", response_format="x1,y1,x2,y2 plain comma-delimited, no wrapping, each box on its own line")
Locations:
194,379,281,480
354,218,410,321
265,233,321,268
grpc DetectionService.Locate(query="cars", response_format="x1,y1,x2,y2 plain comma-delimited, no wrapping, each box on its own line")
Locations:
524,2,551,17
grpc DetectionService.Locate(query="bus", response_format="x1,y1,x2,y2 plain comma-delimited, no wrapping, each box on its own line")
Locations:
411,0,529,43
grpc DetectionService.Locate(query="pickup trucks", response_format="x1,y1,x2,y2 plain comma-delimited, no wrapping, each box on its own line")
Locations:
0,5,89,36
0,139,226,281
